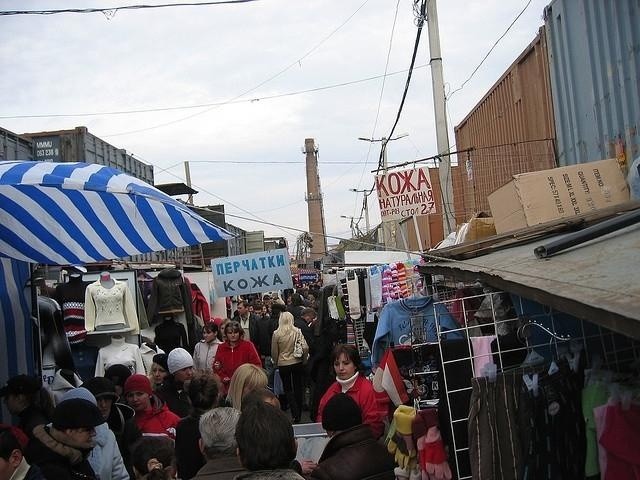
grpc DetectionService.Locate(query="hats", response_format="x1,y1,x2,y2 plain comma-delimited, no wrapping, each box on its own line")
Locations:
167,348,193,375
1,374,43,397
321,392,362,430
50,398,105,429
80,376,120,403
123,374,152,399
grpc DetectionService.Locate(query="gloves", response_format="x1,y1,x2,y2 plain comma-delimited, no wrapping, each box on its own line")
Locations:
335,296,345,318
327,296,339,320
384,405,452,480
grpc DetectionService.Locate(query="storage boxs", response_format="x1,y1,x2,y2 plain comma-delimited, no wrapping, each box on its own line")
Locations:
485,156,630,235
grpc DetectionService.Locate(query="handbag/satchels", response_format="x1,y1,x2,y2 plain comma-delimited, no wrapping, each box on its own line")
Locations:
274,370,286,395
293,343,311,360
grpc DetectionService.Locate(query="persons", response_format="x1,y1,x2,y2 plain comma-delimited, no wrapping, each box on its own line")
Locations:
286,283,322,410
192,322,223,371
316,343,380,440
217,318,231,342
94,335,147,379
271,311,310,424
153,316,188,358
84,271,140,334
139,341,168,379
233,294,289,411
46,368,85,408
310,393,395,479
212,321,264,406
54,273,98,347
1,348,319,479
146,268,194,325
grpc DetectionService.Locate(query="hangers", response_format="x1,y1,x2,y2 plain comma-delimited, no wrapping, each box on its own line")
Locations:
481,323,582,383
